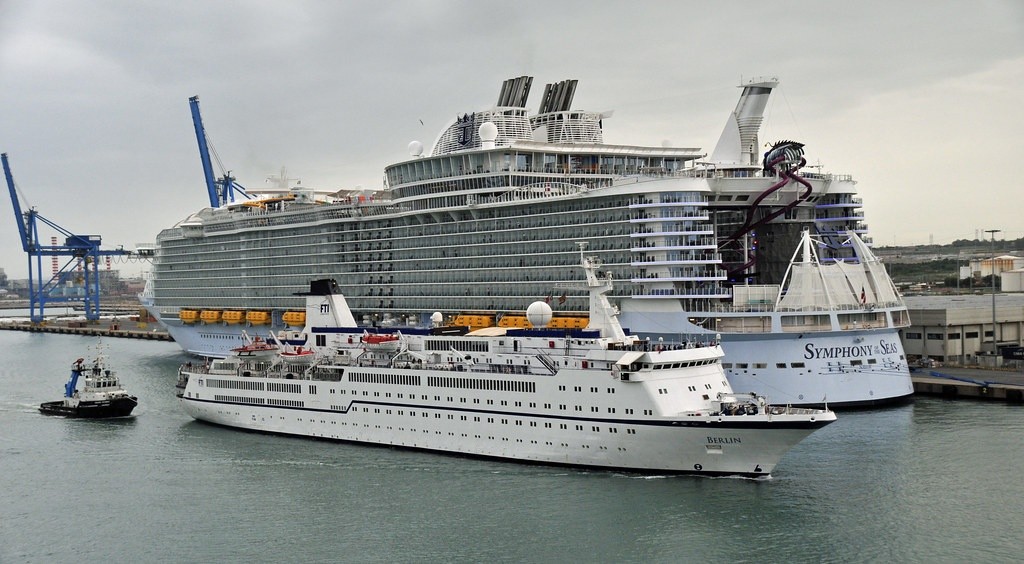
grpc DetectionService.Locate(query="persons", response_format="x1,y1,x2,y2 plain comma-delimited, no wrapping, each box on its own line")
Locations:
189,361,191,372
206,362,211,373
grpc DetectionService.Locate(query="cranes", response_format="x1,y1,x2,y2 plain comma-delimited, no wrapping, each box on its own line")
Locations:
1,153,137,324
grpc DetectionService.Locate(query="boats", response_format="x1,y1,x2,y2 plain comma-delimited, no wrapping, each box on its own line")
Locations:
38,333,137,422
173,277,839,481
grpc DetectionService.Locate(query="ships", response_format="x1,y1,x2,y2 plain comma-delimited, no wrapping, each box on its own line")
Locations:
141,76,917,407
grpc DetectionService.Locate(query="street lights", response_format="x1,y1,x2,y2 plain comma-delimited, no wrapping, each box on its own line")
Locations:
985,230,1002,355
